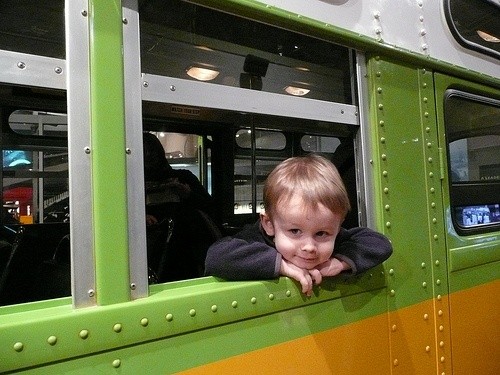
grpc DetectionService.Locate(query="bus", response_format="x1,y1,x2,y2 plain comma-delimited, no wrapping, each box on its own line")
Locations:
1,0,500,375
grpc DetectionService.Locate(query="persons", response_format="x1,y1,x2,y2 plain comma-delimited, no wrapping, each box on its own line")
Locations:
204,155,393,297
143,133,208,224
